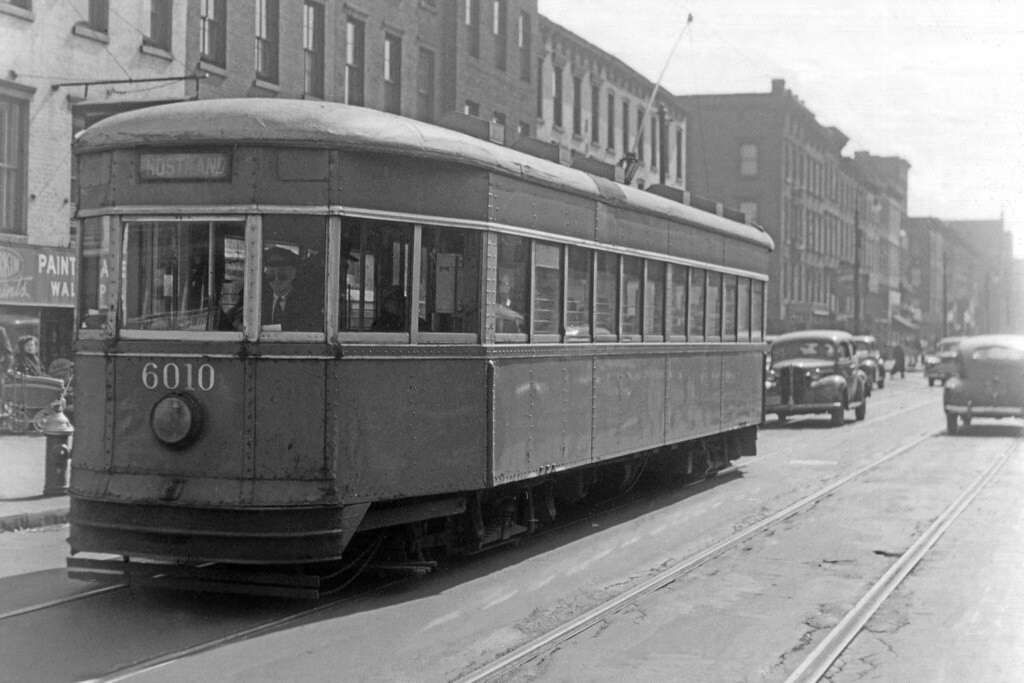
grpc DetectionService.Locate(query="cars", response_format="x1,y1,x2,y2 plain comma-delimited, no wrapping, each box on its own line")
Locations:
924,333,967,386
853,337,885,392
943,336,1024,430
765,330,865,425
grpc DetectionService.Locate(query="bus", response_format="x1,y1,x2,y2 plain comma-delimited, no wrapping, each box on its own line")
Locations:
65,97,781,603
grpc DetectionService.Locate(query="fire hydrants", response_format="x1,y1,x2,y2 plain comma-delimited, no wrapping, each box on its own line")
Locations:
43,400,73,497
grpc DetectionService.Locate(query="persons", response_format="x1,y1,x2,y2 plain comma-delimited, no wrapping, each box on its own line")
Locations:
890,342,906,378
0,327,14,385
379,285,432,333
264,247,324,332
13,334,47,378
279,236,358,333
905,336,928,366
814,343,829,354
